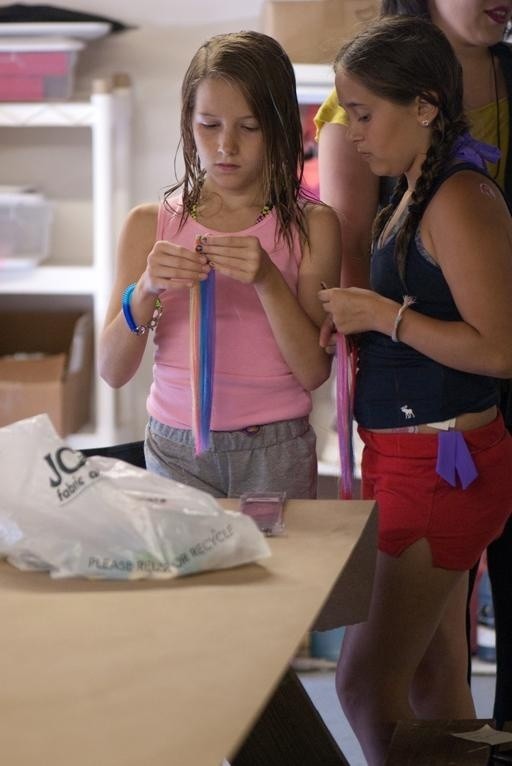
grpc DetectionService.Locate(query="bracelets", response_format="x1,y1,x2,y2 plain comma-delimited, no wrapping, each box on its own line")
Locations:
389,295,417,343
123,283,162,336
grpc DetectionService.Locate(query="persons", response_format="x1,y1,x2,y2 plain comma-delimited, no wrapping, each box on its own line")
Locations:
101,31,342,498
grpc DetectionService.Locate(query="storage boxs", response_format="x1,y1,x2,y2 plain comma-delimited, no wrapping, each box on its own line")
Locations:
264,3,385,66
0,309,91,439
2,35,86,102
0,187,52,269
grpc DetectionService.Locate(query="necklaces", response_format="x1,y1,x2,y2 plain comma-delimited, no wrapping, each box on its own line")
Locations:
463,52,501,183
189,201,275,225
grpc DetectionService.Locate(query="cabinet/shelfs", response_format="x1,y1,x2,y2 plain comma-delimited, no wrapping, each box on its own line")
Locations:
1,72,138,445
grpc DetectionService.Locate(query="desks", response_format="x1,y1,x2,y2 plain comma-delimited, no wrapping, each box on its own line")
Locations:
2,491,378,766
